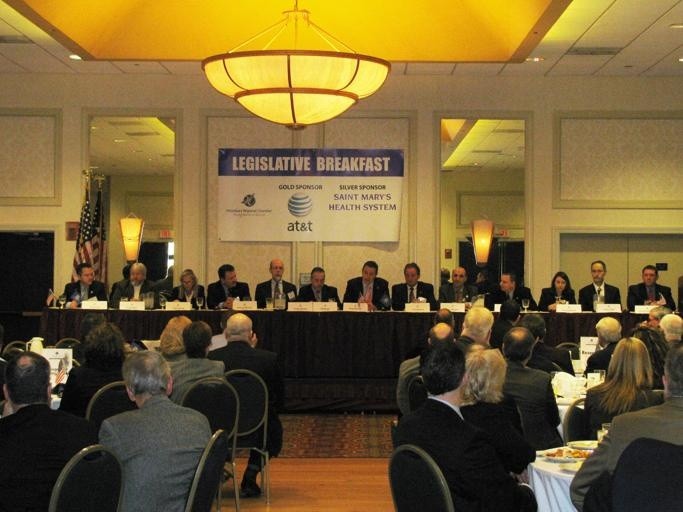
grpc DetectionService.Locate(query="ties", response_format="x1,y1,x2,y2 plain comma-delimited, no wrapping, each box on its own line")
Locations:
409,288,415,302
274,283,282,296
647,289,654,302
597,288,602,295
365,286,372,302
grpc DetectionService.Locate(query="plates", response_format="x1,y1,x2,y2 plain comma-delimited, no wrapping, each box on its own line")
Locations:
541,439,598,473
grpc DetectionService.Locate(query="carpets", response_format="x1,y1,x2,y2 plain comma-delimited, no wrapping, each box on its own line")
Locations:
228,414,400,459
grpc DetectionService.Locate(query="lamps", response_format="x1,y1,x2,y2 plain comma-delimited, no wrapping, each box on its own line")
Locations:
472,219,495,268
201,1,390,131
119,211,144,264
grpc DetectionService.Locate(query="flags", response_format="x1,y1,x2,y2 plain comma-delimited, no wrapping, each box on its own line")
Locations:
70,186,92,274
86,192,111,285
46,290,54,306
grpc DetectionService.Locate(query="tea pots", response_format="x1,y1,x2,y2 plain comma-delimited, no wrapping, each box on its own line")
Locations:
25,336,44,352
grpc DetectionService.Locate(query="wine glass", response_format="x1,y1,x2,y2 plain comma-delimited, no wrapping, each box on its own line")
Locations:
59,295,67,309
196,297,203,310
521,299,530,311
159,298,166,309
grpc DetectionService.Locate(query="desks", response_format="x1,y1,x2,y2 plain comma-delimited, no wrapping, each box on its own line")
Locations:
22,309,661,415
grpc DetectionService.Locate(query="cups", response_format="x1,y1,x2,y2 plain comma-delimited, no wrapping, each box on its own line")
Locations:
120,296,128,301
570,358,585,375
265,298,273,308
587,370,607,389
493,303,501,312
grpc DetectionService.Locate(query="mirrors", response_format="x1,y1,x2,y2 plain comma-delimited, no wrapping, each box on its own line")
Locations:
431,110,533,294
82,108,183,298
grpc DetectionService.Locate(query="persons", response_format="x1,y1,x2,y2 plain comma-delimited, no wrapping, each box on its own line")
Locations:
56,263,252,308
95,351,216,510
343,260,537,311
537,260,676,313
565,344,681,511
255,258,342,309
399,304,682,450
396,340,538,508
1,312,286,512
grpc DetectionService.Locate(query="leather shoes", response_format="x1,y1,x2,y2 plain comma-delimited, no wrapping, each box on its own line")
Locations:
241,479,262,497
